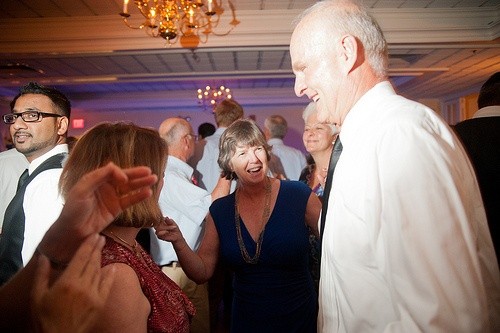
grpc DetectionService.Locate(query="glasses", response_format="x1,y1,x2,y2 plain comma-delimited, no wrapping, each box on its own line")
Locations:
184,133,198,143
2,111,62,124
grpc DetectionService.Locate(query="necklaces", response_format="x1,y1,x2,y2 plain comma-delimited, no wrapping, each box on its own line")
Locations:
235,177,273,264
106,228,137,254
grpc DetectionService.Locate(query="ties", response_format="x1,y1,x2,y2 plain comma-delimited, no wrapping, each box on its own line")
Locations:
192,173,199,187
316,135,343,250
17,168,29,192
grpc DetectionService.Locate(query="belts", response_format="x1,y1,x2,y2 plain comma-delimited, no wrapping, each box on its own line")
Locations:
165,261,181,267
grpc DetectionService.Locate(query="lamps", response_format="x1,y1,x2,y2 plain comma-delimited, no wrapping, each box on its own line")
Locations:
119,0,215,44
197,83,232,111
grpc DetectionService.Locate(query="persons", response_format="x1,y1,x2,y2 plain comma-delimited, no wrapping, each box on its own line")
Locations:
290,0,500,333
0,73,500,333
